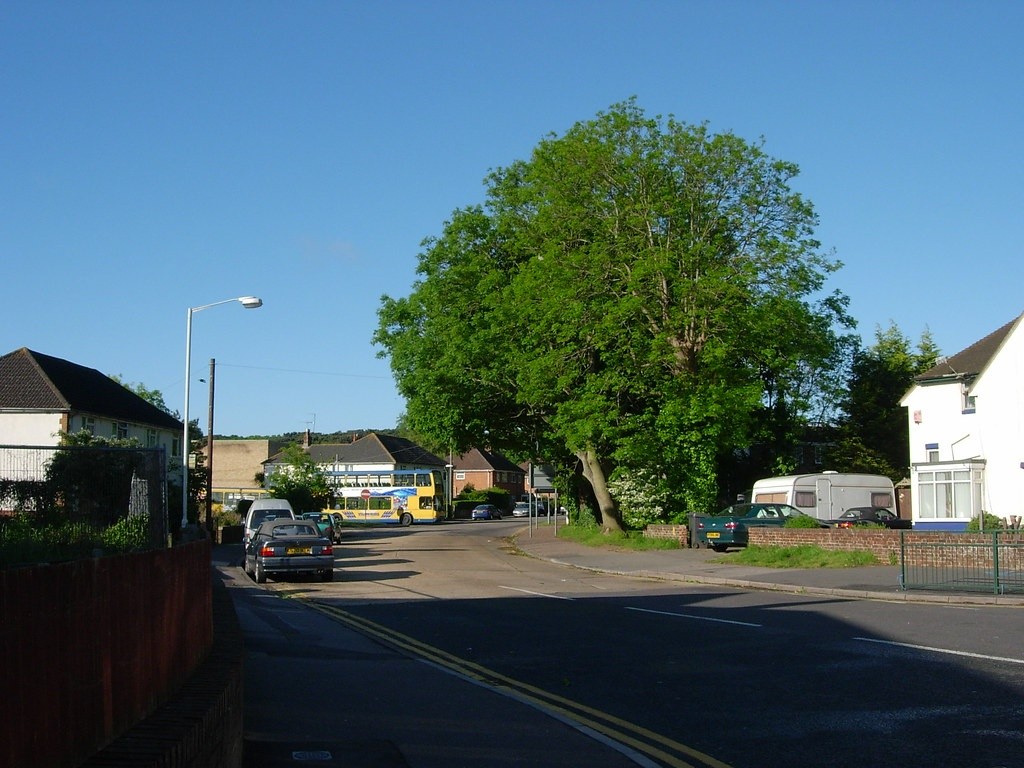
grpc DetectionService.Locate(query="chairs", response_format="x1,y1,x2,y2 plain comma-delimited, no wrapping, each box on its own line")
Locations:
324,481,440,510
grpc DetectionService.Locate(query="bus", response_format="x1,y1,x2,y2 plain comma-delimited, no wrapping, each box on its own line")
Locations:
314,469,446,526
200,487,276,515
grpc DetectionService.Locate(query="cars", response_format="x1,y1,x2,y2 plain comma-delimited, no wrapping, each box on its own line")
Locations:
697,503,824,552
243,520,335,583
472,504,503,520
560,506,566,515
302,512,341,545
513,503,540,517
820,506,912,530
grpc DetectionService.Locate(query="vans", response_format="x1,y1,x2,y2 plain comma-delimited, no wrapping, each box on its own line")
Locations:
241,499,297,551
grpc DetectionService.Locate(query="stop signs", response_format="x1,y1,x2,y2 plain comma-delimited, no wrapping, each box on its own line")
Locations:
360,490,371,499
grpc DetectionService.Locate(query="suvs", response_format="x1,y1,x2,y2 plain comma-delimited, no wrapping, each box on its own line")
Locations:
532,500,553,516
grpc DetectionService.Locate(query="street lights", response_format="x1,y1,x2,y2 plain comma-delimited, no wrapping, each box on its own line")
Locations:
182,296,263,528
